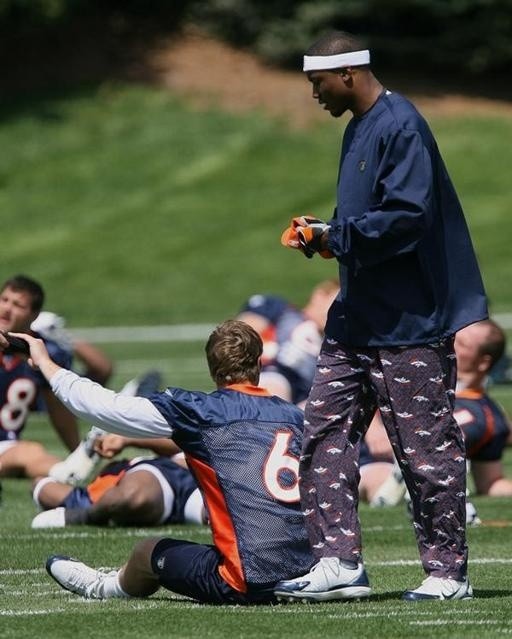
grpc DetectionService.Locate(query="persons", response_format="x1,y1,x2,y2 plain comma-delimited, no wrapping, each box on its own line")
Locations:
8,320,320,602
236,278,512,528
271,30,490,601
1,276,211,530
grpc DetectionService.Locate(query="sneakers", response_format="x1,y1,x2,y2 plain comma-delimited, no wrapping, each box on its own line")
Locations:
46,556,112,600
31,507,66,529
274,557,371,601
48,441,99,485
402,574,474,600
